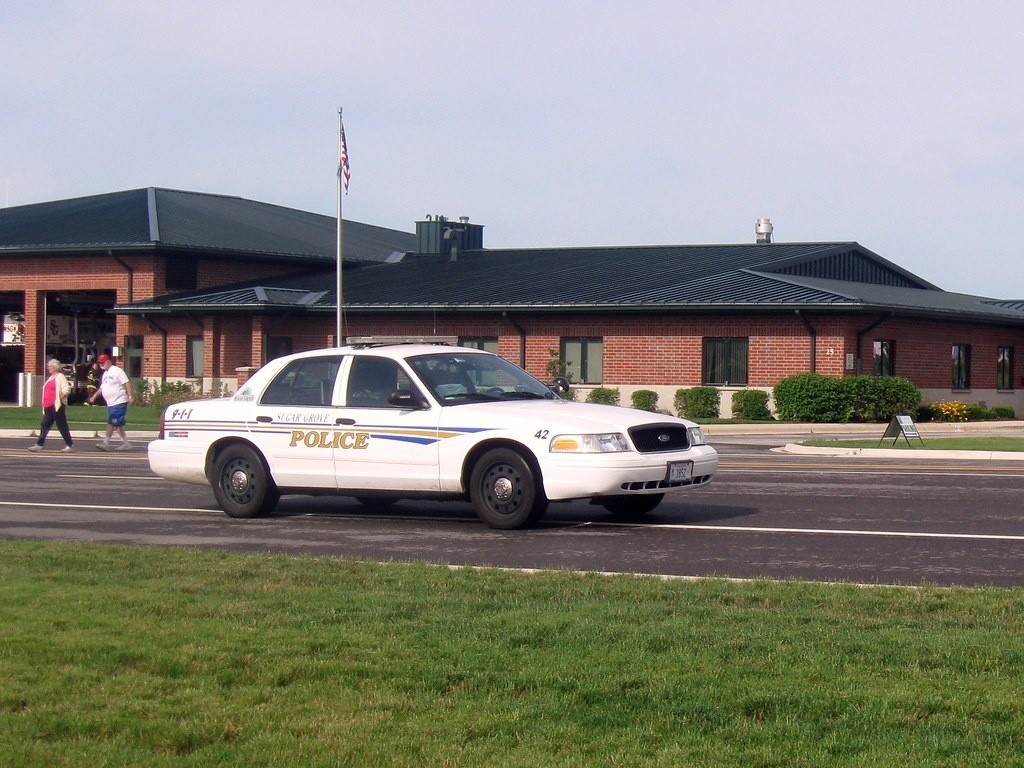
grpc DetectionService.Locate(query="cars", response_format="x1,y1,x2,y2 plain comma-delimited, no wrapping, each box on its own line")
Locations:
147,343,718,529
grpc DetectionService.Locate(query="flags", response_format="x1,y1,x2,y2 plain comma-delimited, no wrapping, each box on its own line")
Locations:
339,118,350,195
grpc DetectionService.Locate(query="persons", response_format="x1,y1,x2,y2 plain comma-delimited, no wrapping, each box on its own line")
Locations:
26,359,77,453
90,353,133,451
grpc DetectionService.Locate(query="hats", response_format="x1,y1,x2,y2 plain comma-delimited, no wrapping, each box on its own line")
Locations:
96,354,109,363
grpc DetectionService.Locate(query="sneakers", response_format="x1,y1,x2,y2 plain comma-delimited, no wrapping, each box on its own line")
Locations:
28,444,43,451
61,445,74,453
96,442,109,451
115,443,132,450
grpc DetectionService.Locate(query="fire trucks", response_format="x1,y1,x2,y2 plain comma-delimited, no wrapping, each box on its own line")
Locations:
0,310,116,405
